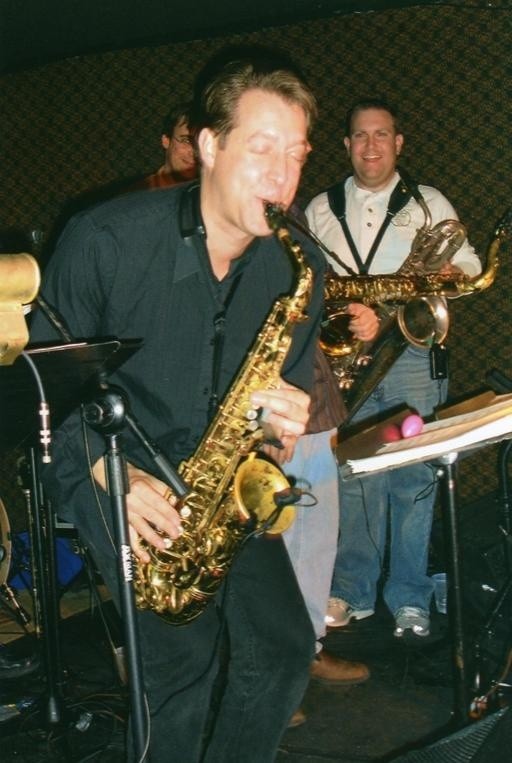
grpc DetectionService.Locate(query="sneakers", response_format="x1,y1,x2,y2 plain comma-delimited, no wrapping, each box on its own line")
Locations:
322,596,375,628
393,604,432,642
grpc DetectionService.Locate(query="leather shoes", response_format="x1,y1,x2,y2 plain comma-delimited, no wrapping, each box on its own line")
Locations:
286,708,307,728
308,646,371,686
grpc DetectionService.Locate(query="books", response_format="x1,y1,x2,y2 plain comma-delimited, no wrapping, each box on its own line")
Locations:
346,393,512,474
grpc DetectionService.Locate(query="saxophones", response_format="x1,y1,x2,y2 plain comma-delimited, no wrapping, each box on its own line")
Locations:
316,209,511,356
334,165,468,432
132,200,315,626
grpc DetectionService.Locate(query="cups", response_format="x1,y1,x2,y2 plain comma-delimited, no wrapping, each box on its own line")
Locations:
115,646,126,683
431,571,449,616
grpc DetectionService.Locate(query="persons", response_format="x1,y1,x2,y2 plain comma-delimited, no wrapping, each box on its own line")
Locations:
301,95,484,644
263,205,372,729
3,46,330,763
135,102,198,192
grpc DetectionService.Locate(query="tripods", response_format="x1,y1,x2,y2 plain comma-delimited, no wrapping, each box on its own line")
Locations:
0,446,129,763
